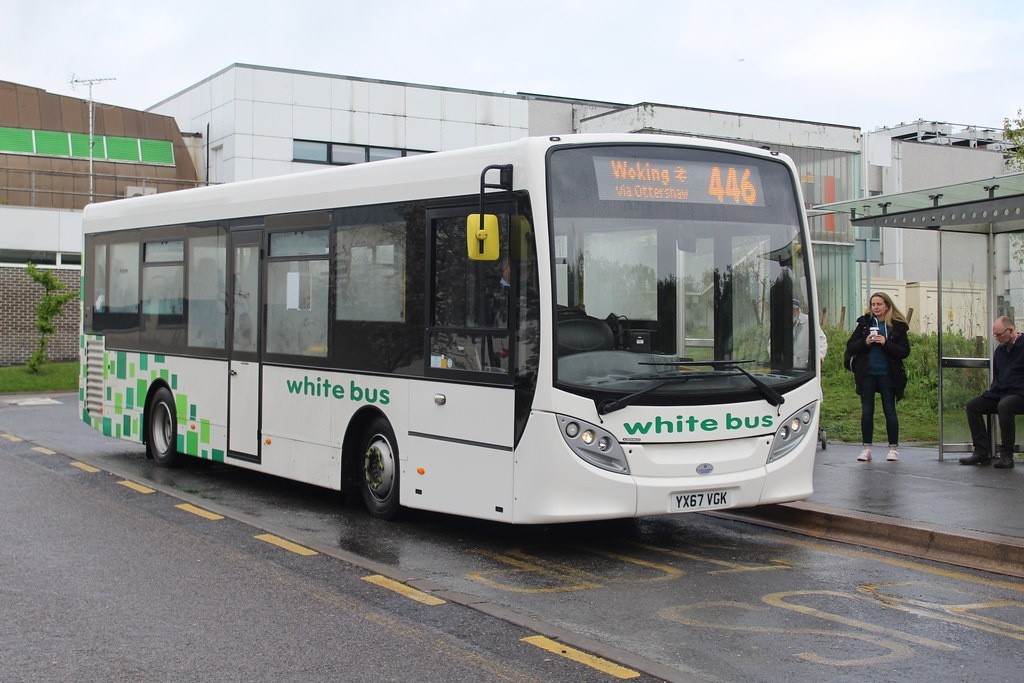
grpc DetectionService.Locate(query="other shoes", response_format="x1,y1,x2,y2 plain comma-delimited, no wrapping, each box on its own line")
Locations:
858,450,873,460
886,450,900,460
994,456,1015,468
959,451,992,466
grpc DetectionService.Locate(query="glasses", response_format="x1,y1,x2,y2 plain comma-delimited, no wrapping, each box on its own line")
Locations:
993,328,1011,336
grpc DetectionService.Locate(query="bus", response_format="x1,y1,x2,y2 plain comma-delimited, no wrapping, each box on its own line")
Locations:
79,133,821,524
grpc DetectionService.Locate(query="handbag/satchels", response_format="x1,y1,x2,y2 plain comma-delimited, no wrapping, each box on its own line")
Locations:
844,351,857,373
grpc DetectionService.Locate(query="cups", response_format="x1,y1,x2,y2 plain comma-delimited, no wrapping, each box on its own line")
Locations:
869,326,879,339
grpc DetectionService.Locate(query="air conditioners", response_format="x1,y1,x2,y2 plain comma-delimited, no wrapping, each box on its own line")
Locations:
124,185,158,198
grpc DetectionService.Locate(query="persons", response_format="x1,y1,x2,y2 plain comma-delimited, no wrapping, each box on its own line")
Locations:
767,299,828,371
844,292,911,461
959,316,1024,468
488,253,538,329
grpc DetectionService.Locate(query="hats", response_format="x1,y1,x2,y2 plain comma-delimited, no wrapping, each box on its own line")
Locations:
792,300,800,309
